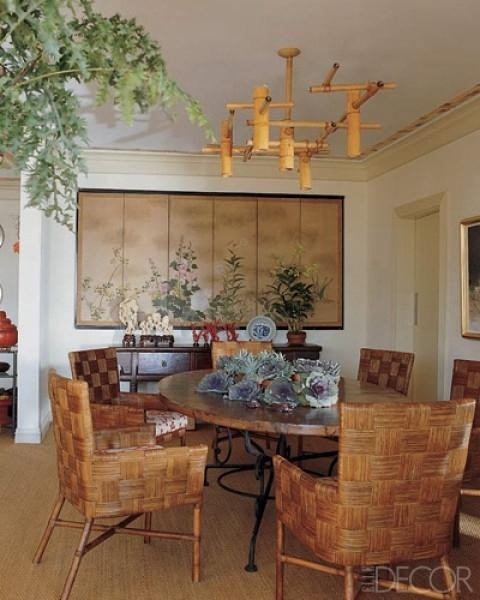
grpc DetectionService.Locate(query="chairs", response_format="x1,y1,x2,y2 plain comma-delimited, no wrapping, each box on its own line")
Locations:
35,341,480,600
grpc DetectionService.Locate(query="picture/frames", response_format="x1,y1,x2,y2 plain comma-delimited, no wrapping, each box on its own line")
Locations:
74,187,345,332
458,215,480,339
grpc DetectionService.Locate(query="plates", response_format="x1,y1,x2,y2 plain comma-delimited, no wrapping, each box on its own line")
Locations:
247,315,276,341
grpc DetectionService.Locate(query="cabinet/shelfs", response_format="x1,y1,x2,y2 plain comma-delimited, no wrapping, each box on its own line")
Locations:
0,349,18,438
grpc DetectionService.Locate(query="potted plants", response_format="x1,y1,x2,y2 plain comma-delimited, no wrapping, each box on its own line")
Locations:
257,263,317,345
